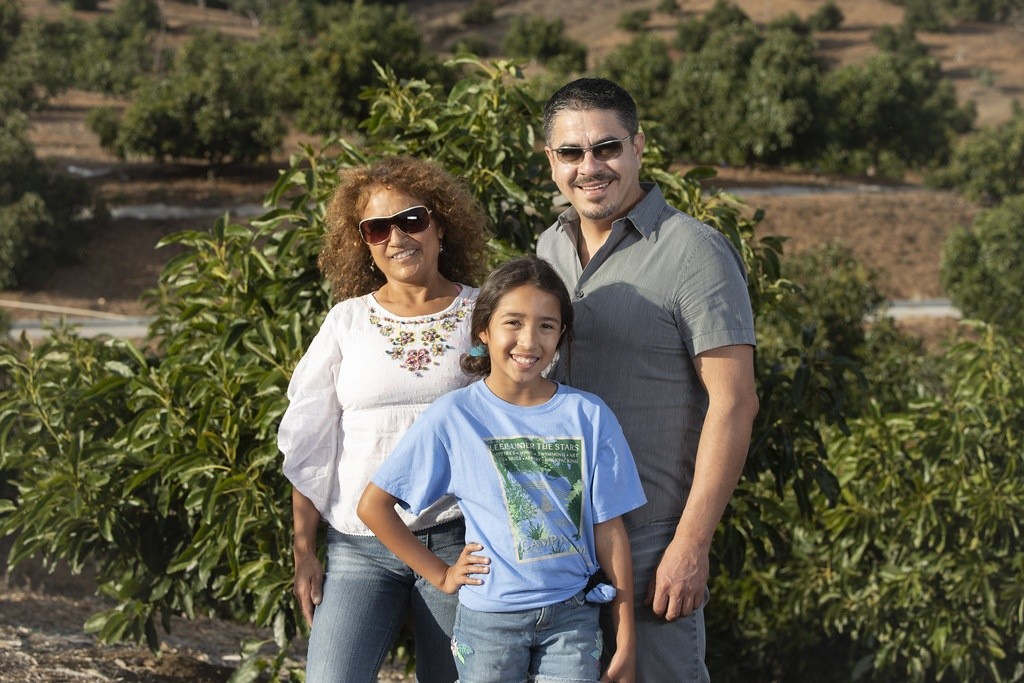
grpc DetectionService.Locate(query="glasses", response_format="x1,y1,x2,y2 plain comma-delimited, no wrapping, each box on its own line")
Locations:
357,206,434,246
551,134,633,165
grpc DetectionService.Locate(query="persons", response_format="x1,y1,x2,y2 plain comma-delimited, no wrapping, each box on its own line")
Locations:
278,156,485,683
357,257,647,682
535,78,759,683
